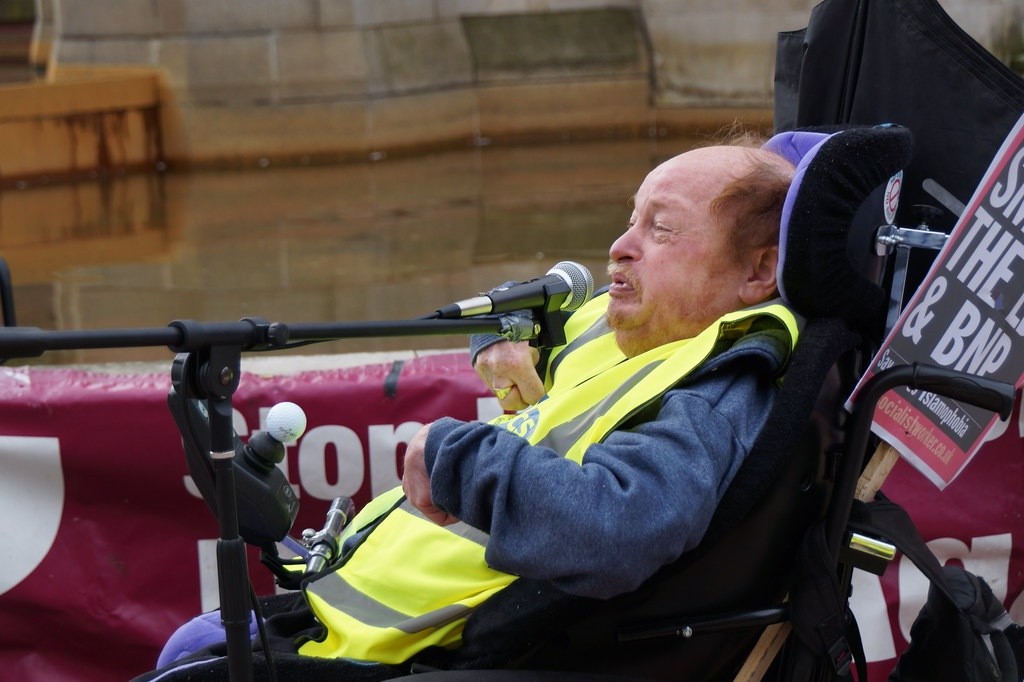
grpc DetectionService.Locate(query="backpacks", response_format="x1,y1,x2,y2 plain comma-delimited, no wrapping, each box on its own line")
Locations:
794,484,1024,682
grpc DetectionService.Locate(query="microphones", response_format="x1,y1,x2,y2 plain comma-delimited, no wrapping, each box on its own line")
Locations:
435,261,593,318
305,496,355,579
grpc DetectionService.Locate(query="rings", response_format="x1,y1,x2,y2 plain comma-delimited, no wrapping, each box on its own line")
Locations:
493,384,516,401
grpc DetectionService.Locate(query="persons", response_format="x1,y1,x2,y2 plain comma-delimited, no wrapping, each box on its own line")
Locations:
155,118,801,668
